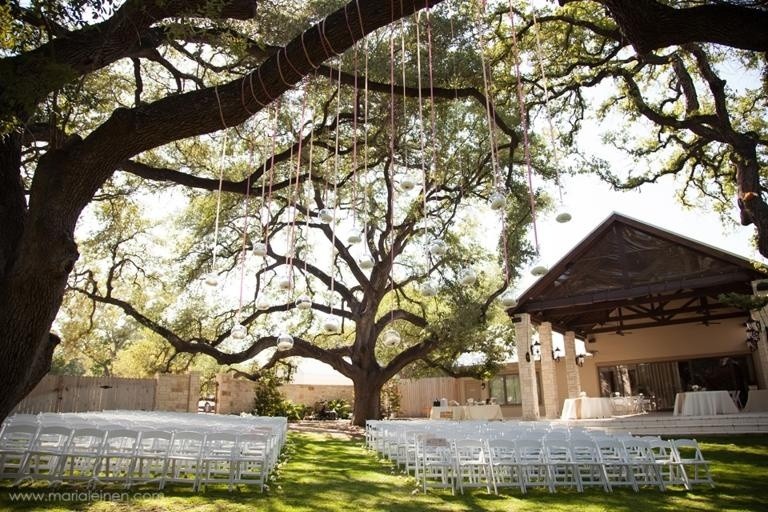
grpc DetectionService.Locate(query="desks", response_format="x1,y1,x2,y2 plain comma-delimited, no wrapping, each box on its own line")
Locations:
429,404,503,418
562,397,611,419
672,390,768,415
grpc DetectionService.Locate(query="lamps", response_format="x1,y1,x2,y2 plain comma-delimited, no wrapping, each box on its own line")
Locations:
526,341,542,363
575,353,585,368
742,315,762,351
552,346,561,362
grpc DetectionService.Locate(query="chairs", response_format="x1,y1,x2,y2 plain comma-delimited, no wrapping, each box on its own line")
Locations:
364,417,716,499
1,408,289,493
